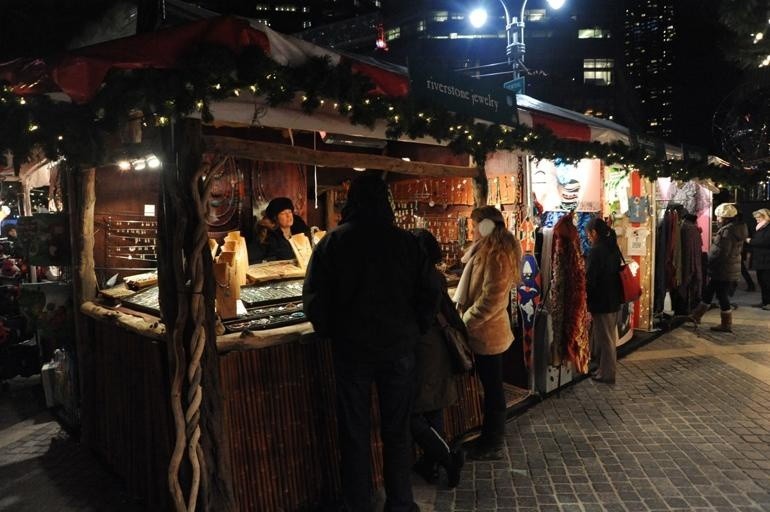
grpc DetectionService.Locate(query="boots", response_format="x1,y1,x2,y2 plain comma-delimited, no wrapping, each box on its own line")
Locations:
708,311,733,334
692,300,711,321
411,427,467,489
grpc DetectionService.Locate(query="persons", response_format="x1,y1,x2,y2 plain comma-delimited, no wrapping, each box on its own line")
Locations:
3,221,18,241
453,205,524,464
584,217,625,385
407,228,474,489
745,207,770,313
261,196,313,260
679,212,702,300
301,168,436,512
688,201,749,333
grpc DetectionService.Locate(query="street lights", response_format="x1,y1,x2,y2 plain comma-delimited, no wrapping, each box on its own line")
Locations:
469,0,566,80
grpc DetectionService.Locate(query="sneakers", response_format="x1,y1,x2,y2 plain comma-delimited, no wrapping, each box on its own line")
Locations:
749,302,770,310
460,432,506,464
587,367,617,386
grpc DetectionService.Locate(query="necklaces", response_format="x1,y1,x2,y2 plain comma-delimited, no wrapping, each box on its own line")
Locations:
238,281,304,304
234,259,237,275
292,238,309,262
199,154,240,230
393,177,524,249
249,159,305,219
215,269,233,297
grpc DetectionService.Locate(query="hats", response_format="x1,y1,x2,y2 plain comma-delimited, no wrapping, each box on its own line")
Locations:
265,195,295,220
752,207,770,233
713,201,739,219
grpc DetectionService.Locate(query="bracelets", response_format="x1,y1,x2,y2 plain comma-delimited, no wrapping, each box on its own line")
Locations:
229,303,308,330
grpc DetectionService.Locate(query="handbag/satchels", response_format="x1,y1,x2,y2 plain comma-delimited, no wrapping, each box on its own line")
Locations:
615,243,644,305
442,319,477,378
